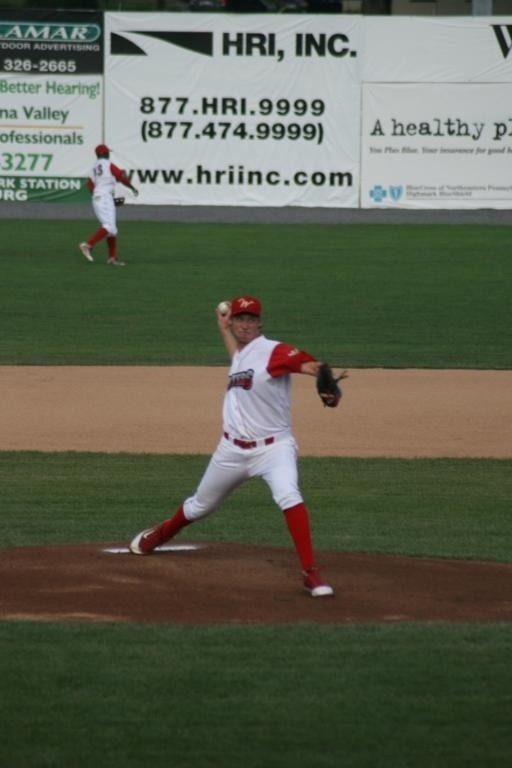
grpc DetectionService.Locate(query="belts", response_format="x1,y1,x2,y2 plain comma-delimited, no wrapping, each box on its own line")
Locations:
223,431,275,449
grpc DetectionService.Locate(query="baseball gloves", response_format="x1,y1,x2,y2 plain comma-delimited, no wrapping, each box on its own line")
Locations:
316,362,348,407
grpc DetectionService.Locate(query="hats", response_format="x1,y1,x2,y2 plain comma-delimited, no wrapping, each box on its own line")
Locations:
95,144,114,154
228,296,262,319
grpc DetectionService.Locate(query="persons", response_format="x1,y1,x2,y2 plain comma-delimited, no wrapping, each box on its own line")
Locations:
77,144,139,268
129,293,342,598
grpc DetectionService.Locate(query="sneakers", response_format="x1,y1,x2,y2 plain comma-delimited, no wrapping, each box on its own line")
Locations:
79,241,94,262
300,566,334,597
106,256,126,267
130,517,179,555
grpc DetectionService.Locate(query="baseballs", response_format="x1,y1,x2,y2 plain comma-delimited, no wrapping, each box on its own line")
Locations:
219,302,229,314
